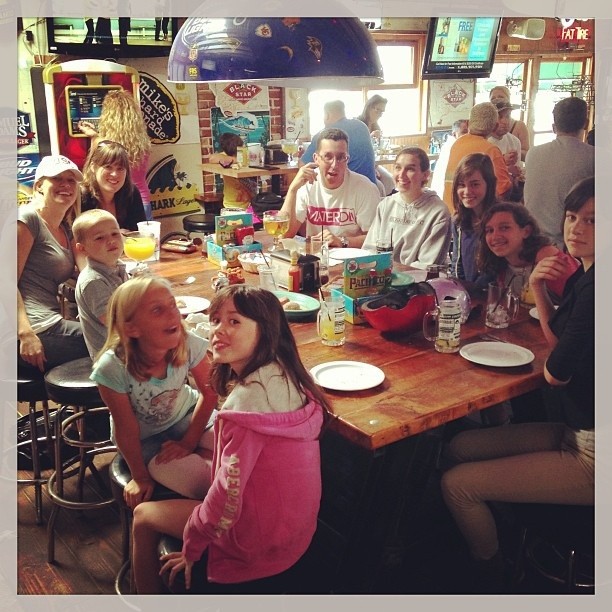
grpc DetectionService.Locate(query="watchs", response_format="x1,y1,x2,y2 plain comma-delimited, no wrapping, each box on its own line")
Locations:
338,235,348,248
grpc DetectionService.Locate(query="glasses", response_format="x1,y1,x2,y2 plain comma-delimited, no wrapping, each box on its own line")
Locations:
318,154,350,165
494,102,512,108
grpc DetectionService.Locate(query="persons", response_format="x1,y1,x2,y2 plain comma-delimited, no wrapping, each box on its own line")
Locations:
485,203,582,303
448,153,509,291
274,130,380,247
524,97,594,251
78,91,152,222
442,178,593,572
298,100,376,186
208,132,264,226
357,94,396,198
90,277,219,512
431,119,469,201
487,105,521,172
72,208,130,361
133,285,325,595
586,131,594,145
82,140,149,232
443,103,516,216
362,149,452,271
489,87,530,158
17,155,87,374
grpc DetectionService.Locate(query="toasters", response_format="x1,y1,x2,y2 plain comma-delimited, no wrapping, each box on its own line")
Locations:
268,247,322,292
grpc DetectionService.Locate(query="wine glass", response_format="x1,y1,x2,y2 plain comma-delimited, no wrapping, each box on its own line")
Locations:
263,211,290,253
123,236,156,273
288,89,300,109
281,139,299,167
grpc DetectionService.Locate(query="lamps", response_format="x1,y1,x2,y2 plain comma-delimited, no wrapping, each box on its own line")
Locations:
165,2,383,90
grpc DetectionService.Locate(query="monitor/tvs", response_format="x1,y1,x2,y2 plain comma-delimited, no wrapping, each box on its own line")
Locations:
419,18,503,80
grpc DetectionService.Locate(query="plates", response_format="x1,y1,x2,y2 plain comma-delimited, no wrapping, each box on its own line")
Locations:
459,340,536,369
329,247,371,260
310,359,384,392
399,269,447,285
528,306,560,321
271,291,320,320
174,295,211,315
389,272,415,287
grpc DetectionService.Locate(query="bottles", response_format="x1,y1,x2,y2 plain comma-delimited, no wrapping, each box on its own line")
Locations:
288,253,300,293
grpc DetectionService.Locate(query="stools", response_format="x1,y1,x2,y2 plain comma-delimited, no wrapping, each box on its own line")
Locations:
252,194,286,210
2,369,82,521
110,452,176,610
46,356,132,563
492,504,595,595
160,534,208,596
183,214,217,238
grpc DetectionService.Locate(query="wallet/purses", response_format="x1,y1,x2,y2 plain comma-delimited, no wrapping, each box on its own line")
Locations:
161,239,196,254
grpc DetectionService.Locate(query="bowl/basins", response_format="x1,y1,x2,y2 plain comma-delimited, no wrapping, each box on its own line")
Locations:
237,253,273,274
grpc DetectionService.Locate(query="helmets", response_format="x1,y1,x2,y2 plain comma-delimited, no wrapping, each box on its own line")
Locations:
426,278,471,325
361,282,435,340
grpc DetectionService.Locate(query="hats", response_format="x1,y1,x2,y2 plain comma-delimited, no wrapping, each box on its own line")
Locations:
491,97,516,112
33,155,83,192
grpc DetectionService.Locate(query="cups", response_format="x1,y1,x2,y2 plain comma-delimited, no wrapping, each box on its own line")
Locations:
137,221,160,262
316,297,345,346
257,266,277,291
378,139,390,160
422,302,460,355
485,281,519,329
376,239,394,272
310,238,329,274
246,142,261,167
